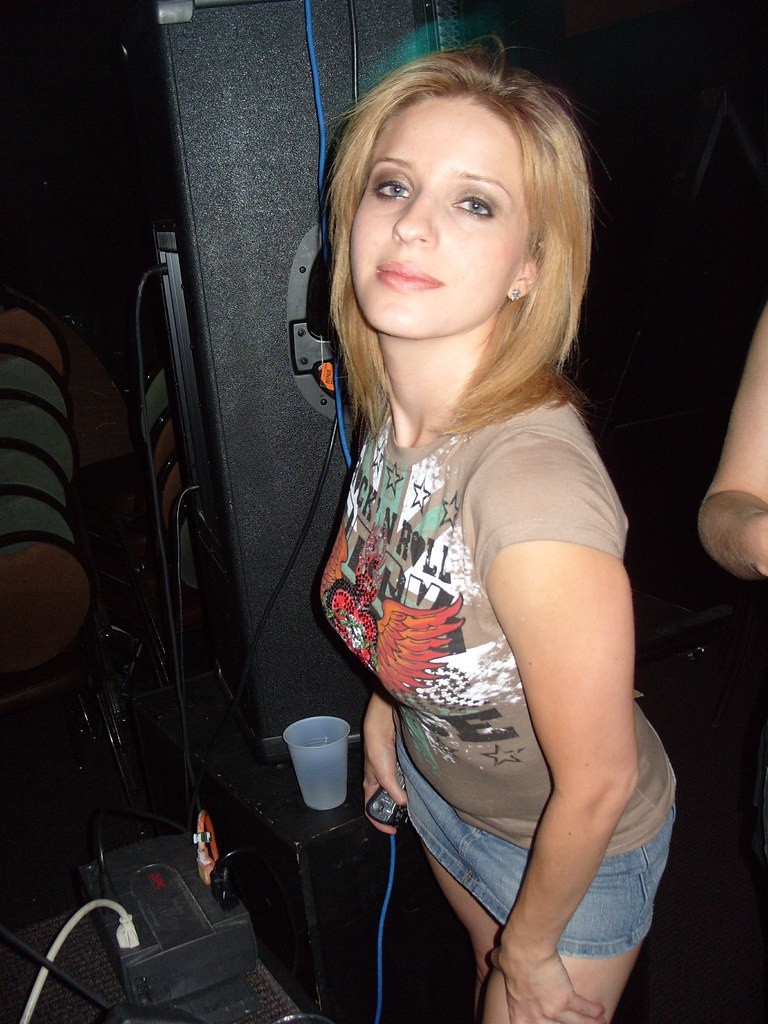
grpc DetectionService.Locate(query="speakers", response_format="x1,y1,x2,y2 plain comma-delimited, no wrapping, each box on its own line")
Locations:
131,0,470,769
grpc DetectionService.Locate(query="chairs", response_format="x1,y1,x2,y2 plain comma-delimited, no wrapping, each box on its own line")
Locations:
0,288,170,823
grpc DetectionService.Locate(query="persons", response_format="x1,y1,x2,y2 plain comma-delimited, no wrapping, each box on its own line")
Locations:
317,49,678,1024
697,302,768,582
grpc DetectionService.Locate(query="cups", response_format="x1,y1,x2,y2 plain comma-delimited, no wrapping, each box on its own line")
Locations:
283,716,350,810
